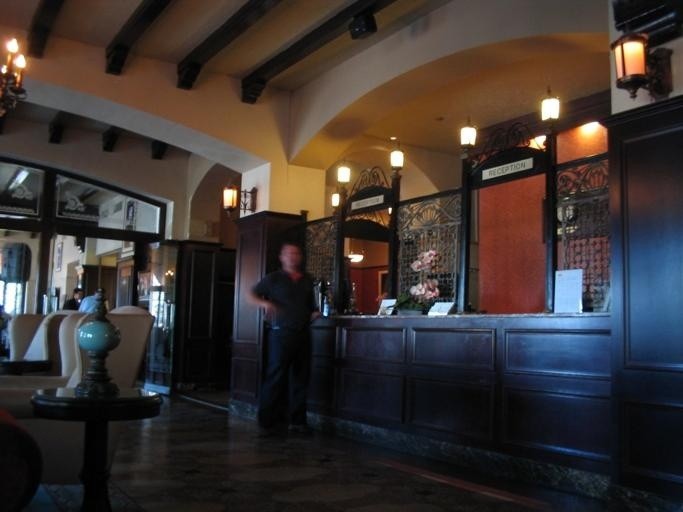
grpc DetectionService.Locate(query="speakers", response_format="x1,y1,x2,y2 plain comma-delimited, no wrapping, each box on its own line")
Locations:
349,15,376,40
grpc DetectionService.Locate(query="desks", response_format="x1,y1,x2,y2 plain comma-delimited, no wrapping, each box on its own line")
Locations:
30,387,163,512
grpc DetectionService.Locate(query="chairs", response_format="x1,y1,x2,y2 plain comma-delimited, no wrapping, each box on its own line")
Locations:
0,305,156,486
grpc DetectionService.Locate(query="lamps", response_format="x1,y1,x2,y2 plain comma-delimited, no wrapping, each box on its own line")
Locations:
390,137,405,173
459,114,477,151
331,186,339,208
611,24,674,100
0,37,28,121
223,179,258,218
540,88,560,125
337,159,351,185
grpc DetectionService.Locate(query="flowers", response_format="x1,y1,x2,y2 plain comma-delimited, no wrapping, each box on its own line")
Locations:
387,249,439,311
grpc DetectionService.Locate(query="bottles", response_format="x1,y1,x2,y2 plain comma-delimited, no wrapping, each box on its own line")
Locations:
323,295,330,316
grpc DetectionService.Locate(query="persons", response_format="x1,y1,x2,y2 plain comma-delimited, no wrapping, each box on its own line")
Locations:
61,287,85,309
76,288,107,314
244,241,323,445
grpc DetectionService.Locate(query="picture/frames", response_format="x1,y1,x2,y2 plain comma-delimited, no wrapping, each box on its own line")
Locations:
122,198,135,253
56,242,63,272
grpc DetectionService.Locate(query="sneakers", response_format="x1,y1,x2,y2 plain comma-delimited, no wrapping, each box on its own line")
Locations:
288,424,313,434
260,430,288,441
